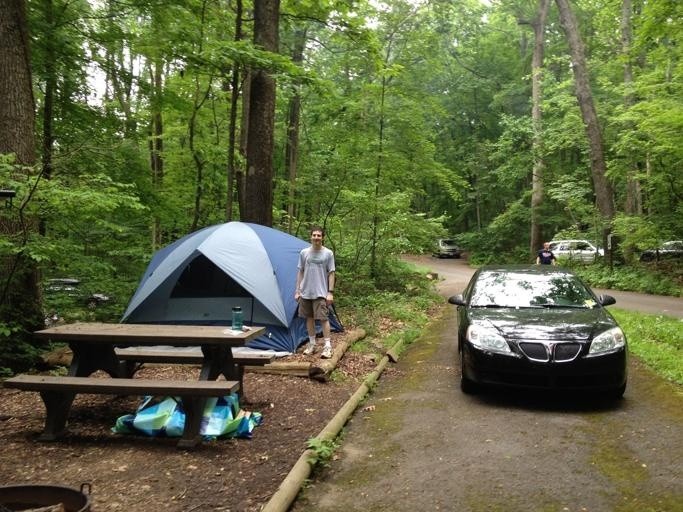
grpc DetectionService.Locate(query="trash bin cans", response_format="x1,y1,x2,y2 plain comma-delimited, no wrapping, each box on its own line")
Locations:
0,482,92,512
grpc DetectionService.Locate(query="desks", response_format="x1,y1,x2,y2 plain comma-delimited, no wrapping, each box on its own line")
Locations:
31,322,268,448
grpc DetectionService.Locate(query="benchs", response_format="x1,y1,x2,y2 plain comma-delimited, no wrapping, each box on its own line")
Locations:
2,346,277,449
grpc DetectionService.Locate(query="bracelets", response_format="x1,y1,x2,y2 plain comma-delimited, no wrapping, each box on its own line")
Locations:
328,290,334,293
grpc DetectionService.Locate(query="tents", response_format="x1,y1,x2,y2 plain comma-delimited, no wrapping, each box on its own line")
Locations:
118,221,345,356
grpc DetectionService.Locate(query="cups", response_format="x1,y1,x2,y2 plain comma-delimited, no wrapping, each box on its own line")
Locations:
232,307,243,330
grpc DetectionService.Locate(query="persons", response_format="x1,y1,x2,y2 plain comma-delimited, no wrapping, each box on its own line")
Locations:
537,242,555,265
294,226,336,359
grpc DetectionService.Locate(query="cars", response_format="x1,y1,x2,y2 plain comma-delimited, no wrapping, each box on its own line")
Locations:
545,240,683,262
432,239,460,258
38,278,115,311
448,264,627,398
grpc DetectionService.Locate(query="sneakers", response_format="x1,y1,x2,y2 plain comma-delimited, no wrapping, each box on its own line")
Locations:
321,346,333,358
303,344,317,354
